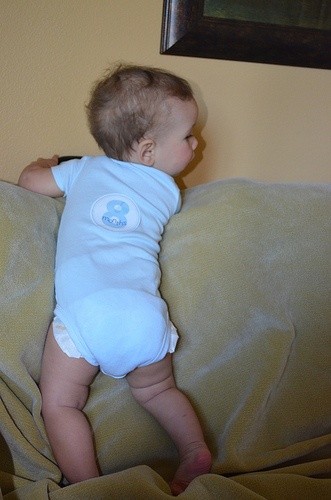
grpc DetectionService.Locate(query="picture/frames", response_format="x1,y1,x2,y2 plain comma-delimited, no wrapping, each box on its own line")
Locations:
159,0,331,71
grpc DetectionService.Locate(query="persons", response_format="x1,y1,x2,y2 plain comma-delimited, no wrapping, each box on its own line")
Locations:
18,66,214,497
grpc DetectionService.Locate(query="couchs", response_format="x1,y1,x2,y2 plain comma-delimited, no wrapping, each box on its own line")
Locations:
1,178,330,500
